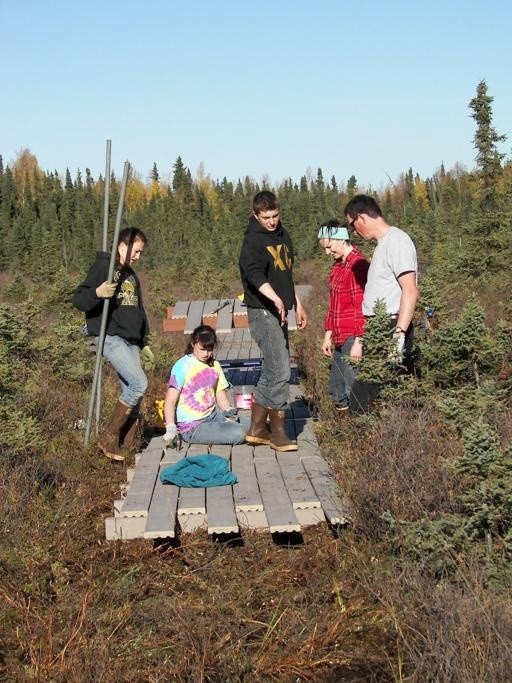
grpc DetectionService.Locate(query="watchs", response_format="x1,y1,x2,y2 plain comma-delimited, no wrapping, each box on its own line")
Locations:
393,326,407,336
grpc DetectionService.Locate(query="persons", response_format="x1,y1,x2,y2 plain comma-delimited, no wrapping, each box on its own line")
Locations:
71,226,160,463
345,194,420,376
314,217,372,420
163,323,250,452
238,190,309,451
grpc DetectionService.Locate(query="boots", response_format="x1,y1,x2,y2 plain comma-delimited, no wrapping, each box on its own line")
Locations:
96,399,126,462
267,407,298,452
245,402,271,444
120,416,143,453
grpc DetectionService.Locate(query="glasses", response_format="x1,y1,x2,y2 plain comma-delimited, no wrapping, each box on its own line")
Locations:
349,216,358,228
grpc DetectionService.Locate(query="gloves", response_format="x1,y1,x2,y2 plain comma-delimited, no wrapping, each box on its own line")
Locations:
140,345,156,372
163,423,179,449
96,281,119,298
386,333,406,364
